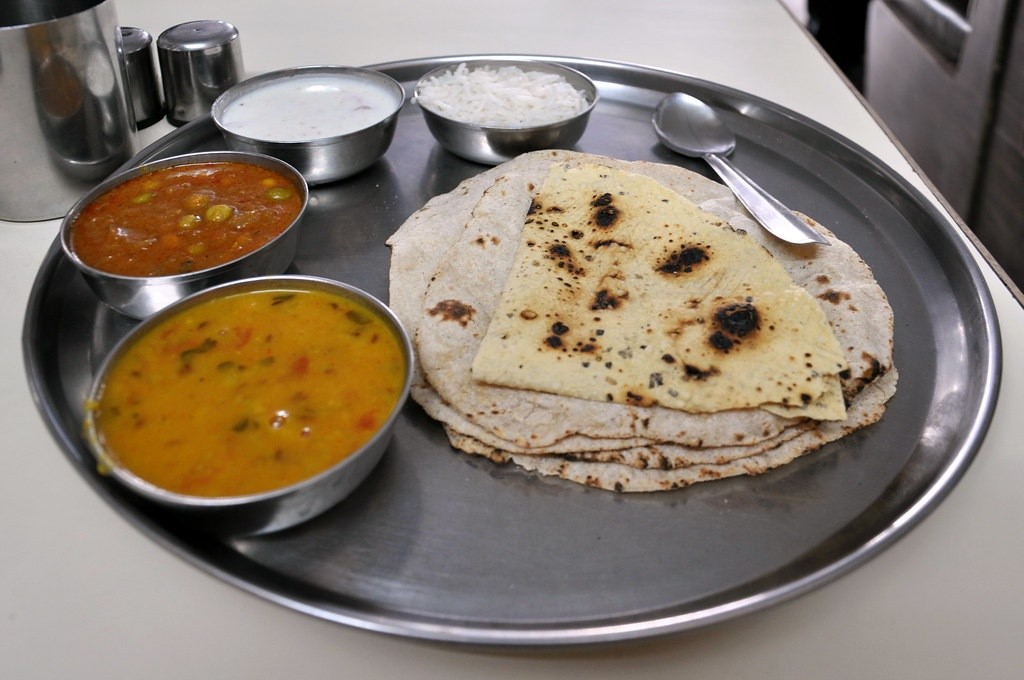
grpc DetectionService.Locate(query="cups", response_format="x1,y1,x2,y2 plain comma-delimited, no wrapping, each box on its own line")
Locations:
158,20,245,127
0,2,136,223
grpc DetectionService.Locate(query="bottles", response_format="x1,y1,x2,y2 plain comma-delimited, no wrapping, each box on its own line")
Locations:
120,27,166,132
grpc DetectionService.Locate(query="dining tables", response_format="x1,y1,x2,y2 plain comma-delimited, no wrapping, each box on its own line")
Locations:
0,1,1023,680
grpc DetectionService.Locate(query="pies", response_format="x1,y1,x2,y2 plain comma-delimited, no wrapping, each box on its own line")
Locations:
385,146,899,495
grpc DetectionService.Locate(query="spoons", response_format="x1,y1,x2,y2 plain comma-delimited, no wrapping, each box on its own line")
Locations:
650,92,832,245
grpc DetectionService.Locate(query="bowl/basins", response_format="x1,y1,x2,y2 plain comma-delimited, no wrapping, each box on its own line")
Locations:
34,55,89,154
209,64,406,189
414,61,601,166
77,37,119,123
59,147,310,323
84,272,417,537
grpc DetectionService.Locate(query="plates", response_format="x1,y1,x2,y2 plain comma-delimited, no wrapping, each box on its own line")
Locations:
17,53,1001,648
